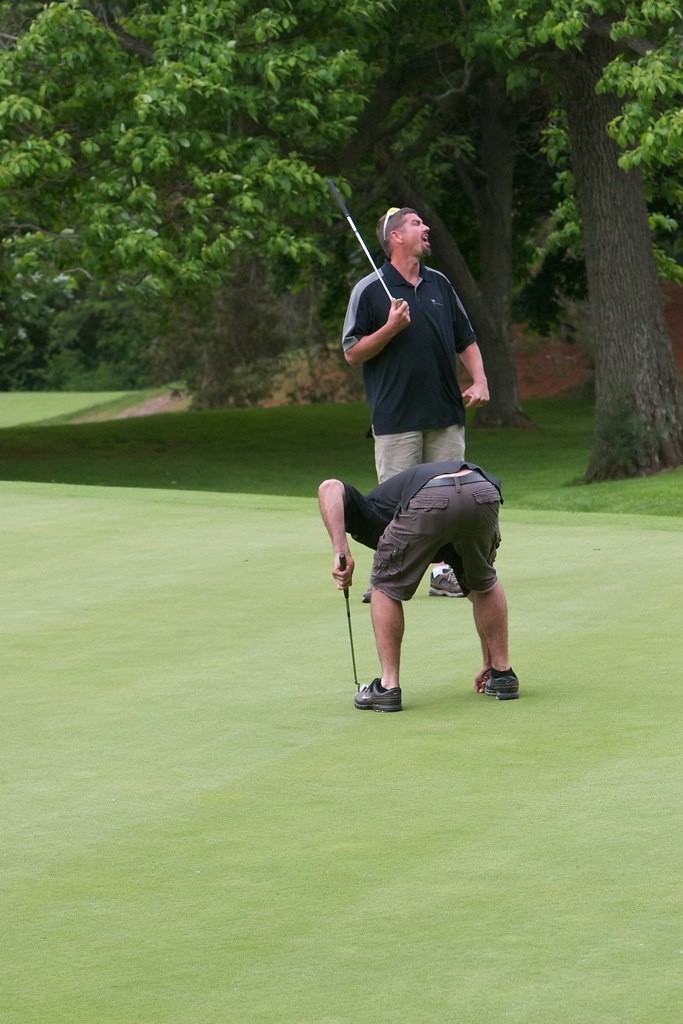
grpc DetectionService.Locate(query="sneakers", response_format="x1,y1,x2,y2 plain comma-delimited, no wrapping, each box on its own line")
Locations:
429,566,466,597
484,666,519,700
353,678,402,713
361,586,372,602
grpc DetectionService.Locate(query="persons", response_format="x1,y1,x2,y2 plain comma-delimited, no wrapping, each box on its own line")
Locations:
341,208,490,603
317,460,520,712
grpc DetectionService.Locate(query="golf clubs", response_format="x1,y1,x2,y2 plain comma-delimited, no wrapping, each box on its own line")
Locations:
327,179,404,309
338,552,368,696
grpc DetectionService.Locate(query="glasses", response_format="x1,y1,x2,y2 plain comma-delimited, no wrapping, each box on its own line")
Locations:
383,207,400,241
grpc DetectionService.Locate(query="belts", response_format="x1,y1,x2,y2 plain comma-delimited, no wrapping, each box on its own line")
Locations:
423,471,488,489
